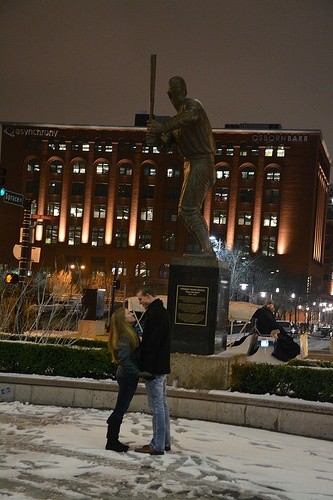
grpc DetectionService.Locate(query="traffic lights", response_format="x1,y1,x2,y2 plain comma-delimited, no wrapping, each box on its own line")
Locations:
0,186,6,197
7,273,19,283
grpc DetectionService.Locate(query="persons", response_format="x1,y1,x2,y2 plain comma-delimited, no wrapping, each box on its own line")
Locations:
104,285,172,455
145,75,219,258
247,299,277,355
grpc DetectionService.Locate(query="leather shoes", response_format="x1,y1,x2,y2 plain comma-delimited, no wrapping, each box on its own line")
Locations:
135,447,164,455
143,444,171,451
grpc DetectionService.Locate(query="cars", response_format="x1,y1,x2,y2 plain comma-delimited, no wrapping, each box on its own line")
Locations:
276,319,295,336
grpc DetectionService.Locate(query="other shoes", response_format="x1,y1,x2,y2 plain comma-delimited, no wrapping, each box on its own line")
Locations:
106,442,130,452
